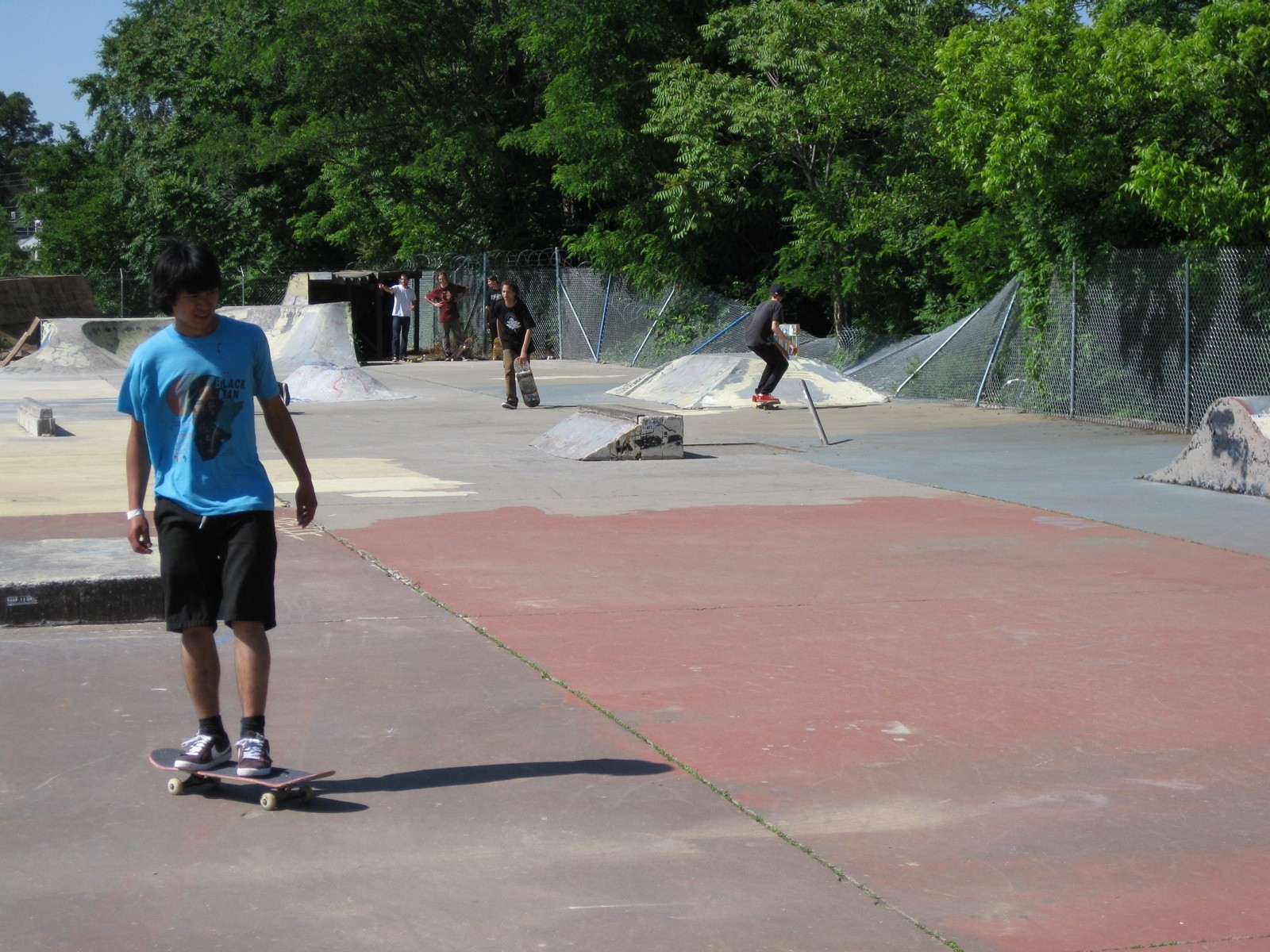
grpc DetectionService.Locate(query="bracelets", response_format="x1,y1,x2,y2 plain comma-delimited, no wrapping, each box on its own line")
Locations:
127,508,144,520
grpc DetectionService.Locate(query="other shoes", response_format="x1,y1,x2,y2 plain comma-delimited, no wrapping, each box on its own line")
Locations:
445,358,451,361
392,357,397,363
399,357,405,362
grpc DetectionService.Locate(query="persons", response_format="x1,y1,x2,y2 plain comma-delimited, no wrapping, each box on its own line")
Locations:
484,274,507,349
378,272,417,361
491,279,536,409
744,284,798,404
424,270,472,361
114,238,317,777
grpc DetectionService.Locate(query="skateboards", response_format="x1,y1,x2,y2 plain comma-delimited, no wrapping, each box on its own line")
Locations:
404,354,423,363
148,747,336,811
492,336,504,361
514,356,540,408
445,336,473,362
755,402,781,410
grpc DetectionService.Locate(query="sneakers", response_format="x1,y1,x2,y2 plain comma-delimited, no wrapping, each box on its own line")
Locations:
174,727,232,771
234,730,273,776
756,395,782,402
502,401,517,409
752,395,757,402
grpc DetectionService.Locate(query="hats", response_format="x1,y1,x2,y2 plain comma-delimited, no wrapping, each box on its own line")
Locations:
771,283,786,296
487,275,500,284
